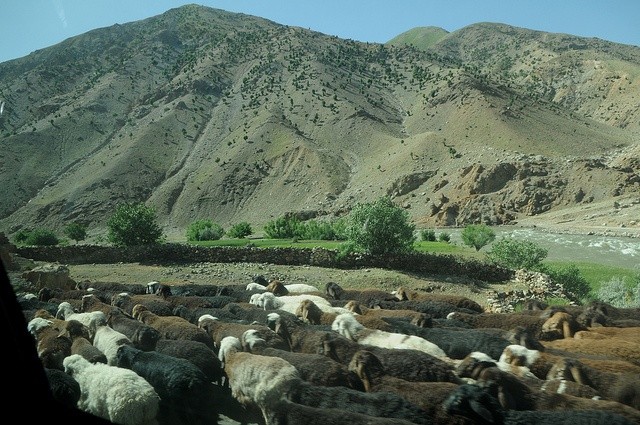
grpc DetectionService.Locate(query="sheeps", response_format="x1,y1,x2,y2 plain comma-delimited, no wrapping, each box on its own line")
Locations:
89,317,131,368
16,280,331,317
219,336,299,425
56,320,107,363
27,316,69,358
198,313,289,354
133,325,221,383
27,316,57,355
132,304,214,350
102,306,139,340
63,354,160,425
116,346,208,425
44,367,80,405
281,281,640,425
